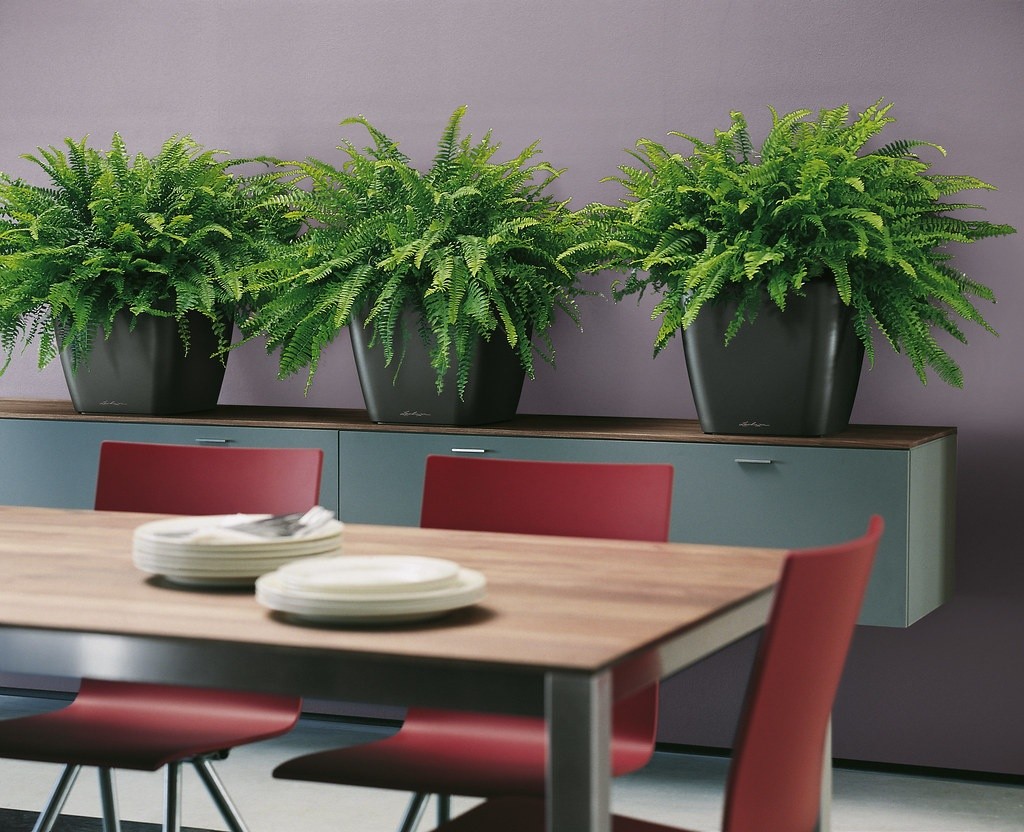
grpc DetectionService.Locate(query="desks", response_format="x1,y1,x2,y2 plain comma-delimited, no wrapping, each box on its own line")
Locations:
0,500,847,832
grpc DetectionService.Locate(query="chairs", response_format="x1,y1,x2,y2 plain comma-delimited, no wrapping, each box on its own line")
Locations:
435,515,883,832
1,438,323,831
271,453,674,832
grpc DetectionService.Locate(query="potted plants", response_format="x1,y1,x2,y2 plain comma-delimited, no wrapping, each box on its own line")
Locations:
0,100,1016,434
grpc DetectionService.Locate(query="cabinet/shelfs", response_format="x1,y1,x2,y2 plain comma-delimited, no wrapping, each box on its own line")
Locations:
0,399,960,625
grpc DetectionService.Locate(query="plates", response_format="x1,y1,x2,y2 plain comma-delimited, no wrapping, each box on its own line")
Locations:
130,513,348,587
254,554,489,630
